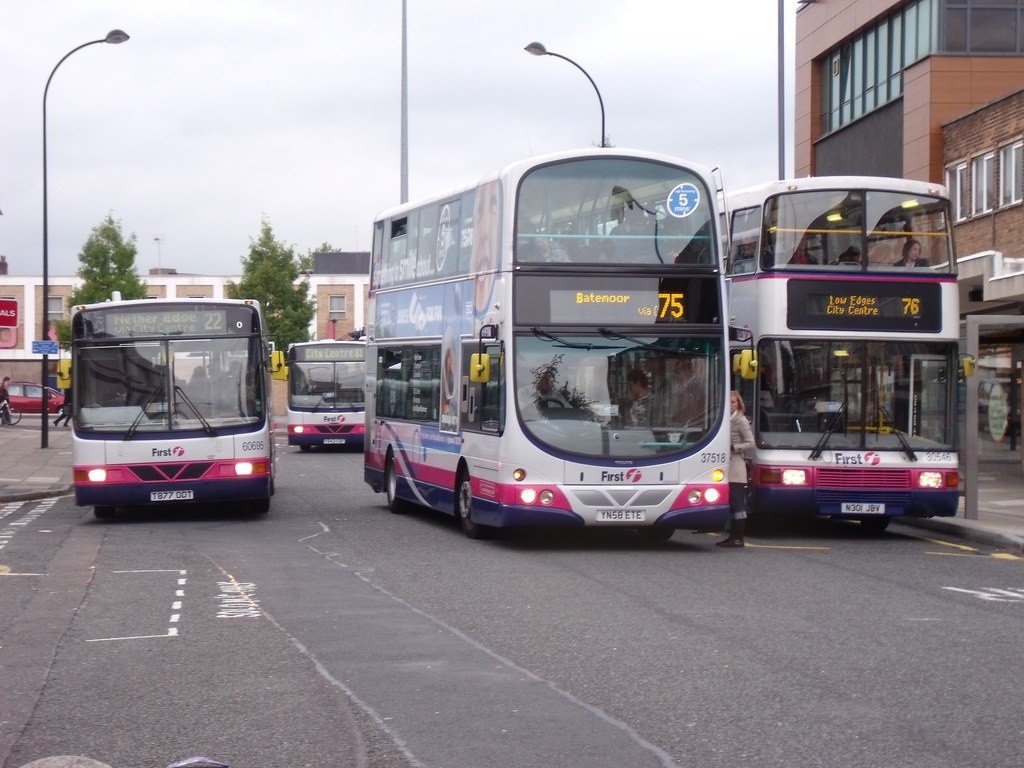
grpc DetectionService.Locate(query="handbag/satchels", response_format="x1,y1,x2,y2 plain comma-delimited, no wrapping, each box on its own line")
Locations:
63,406,71,415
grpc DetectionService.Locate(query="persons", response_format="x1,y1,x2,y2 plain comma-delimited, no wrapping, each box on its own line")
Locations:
301,376,322,394
0,376,10,424
716,389,756,548
627,368,667,427
673,359,706,429
187,360,257,420
54,387,73,427
518,364,574,423
518,217,701,263
762,230,929,269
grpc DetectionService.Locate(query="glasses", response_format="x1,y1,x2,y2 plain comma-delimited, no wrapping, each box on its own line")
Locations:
543,378,555,384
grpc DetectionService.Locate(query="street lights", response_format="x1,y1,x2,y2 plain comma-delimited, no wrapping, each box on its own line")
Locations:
41,28,130,449
524,42,606,148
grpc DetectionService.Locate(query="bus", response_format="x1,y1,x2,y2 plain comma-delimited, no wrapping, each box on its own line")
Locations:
718,174,977,531
69,290,283,519
284,339,367,454
361,147,732,547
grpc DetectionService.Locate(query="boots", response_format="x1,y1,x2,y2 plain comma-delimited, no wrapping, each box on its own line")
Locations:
716,519,746,547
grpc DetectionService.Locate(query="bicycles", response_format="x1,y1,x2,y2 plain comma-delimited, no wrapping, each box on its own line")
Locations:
0,402,22,425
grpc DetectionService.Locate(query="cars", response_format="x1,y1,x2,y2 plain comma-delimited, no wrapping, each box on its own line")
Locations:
4,381,66,416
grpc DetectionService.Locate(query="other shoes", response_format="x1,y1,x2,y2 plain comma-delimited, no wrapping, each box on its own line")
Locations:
63,424,69,427
53,421,58,426
